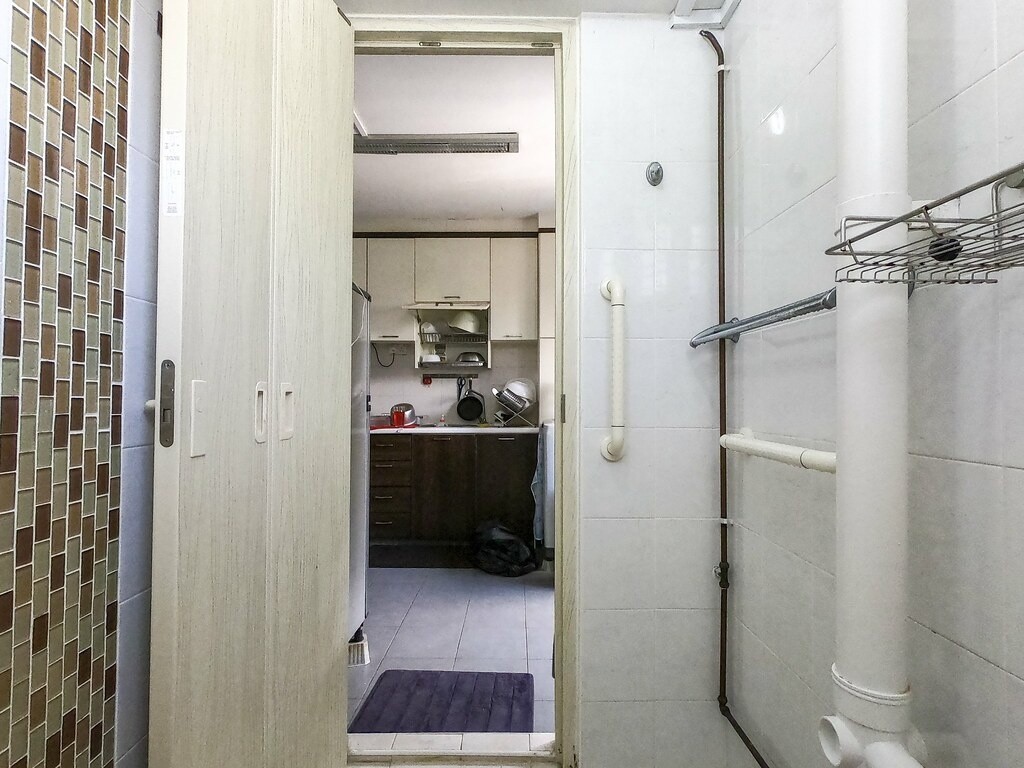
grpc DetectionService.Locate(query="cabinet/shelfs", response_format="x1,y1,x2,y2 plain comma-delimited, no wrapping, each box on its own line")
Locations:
490,238,538,341
412,434,539,541
352,238,368,292
367,238,415,345
401,303,491,368
370,434,412,540
414,238,490,303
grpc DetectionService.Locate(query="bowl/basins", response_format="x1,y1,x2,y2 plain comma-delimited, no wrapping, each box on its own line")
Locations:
422,355,440,362
505,381,533,400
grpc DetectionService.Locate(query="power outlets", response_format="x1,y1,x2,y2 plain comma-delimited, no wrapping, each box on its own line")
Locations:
389,344,407,355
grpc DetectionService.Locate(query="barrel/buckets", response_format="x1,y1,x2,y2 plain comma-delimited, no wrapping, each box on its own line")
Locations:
498,388,526,413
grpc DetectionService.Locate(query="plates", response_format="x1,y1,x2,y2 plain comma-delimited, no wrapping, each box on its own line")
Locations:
504,378,536,402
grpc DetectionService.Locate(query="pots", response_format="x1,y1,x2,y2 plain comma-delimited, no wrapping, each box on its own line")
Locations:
448,311,480,333
455,352,485,362
457,377,484,420
390,403,423,427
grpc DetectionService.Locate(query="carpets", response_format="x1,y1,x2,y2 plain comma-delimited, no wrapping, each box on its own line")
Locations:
347,669,534,733
